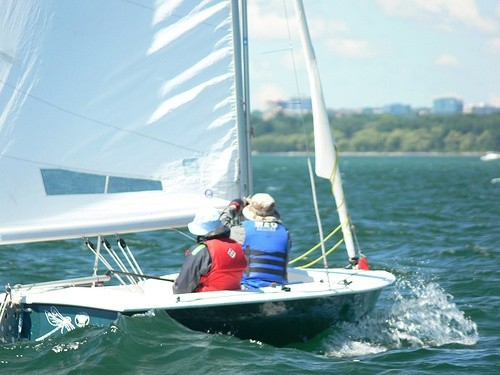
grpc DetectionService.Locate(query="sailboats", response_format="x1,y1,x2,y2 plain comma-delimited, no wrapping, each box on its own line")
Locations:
2,0,397,347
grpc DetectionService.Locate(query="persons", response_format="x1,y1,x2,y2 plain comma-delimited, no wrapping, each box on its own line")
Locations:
219,192,293,290
172,206,249,295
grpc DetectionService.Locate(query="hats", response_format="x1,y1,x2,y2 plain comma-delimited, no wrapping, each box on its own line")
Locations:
186,207,225,236
242,191,281,221
